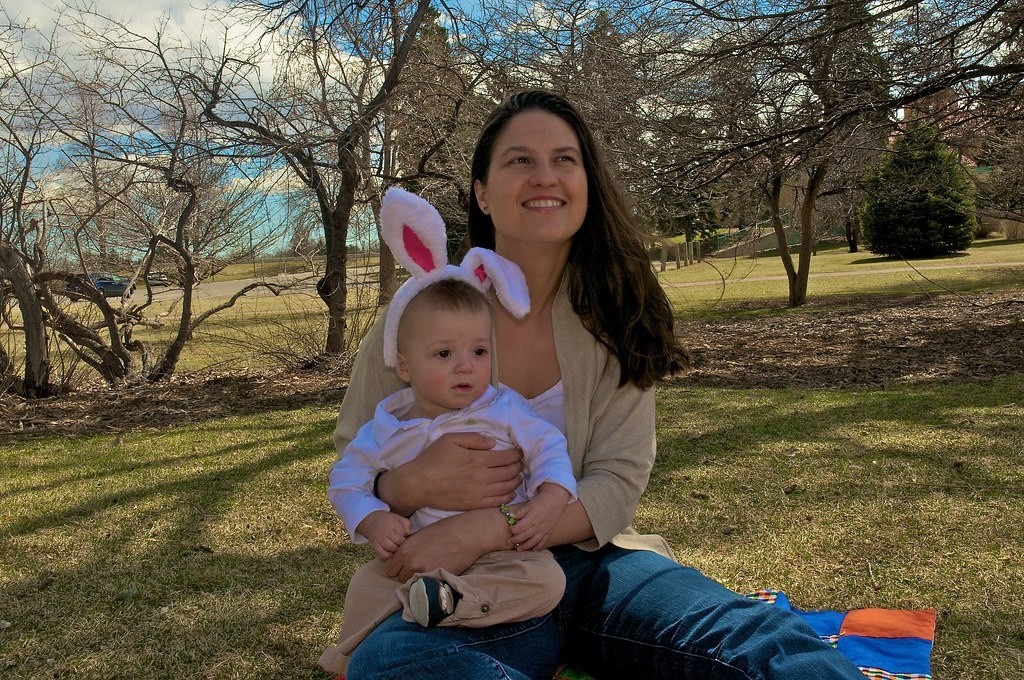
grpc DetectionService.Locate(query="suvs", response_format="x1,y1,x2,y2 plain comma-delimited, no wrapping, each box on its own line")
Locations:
64,271,137,304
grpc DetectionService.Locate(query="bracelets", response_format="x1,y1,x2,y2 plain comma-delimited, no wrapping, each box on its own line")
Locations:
499,504,519,552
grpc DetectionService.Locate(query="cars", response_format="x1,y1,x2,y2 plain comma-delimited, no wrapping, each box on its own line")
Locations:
146,270,168,286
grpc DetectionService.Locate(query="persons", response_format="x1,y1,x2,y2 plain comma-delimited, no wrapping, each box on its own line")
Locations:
326,89,871,680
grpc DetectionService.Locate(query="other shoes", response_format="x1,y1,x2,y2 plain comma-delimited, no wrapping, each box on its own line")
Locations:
409,576,459,628
